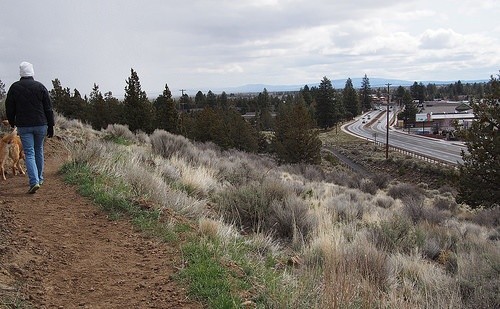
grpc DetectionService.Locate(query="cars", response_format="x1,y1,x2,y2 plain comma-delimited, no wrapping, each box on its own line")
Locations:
361,114,370,124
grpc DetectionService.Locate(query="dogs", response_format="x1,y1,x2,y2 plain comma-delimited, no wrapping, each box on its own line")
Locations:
0,127,26,180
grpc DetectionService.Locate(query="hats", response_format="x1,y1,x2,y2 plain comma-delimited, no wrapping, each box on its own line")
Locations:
19,62,34,77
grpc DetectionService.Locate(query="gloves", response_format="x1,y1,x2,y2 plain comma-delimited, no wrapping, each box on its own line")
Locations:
46,126,54,138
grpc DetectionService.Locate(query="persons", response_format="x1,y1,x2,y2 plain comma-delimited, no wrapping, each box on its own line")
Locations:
5,61,56,195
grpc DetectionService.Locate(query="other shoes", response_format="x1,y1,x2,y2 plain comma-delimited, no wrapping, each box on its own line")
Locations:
27,182,43,193
